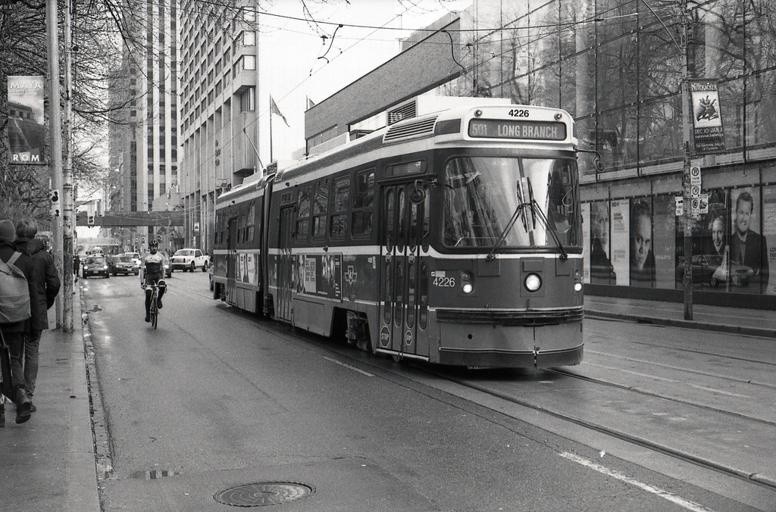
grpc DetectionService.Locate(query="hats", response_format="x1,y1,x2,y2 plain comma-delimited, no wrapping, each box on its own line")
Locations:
0,219,16,242
150,240,160,246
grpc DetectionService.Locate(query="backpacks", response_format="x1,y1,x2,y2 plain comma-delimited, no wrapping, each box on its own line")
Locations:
0,252,33,323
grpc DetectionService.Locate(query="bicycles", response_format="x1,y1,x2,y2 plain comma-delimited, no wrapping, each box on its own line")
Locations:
139,280,169,330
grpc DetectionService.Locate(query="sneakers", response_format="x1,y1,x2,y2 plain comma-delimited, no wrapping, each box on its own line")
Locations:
145,310,151,323
0,404,6,428
157,298,163,310
15,395,36,425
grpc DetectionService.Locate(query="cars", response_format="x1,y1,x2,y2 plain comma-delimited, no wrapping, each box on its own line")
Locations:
142,248,174,279
79,245,142,279
674,254,755,289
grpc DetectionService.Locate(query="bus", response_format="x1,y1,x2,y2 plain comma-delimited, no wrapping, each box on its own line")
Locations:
205,93,588,381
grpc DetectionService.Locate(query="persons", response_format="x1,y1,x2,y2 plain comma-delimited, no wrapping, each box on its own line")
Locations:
589,200,618,286
72,250,80,277
699,215,730,290
629,199,657,287
729,190,772,295
10,216,63,412
0,219,36,427
138,239,167,323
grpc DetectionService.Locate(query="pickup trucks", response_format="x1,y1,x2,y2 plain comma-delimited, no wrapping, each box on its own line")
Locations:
170,248,211,272
